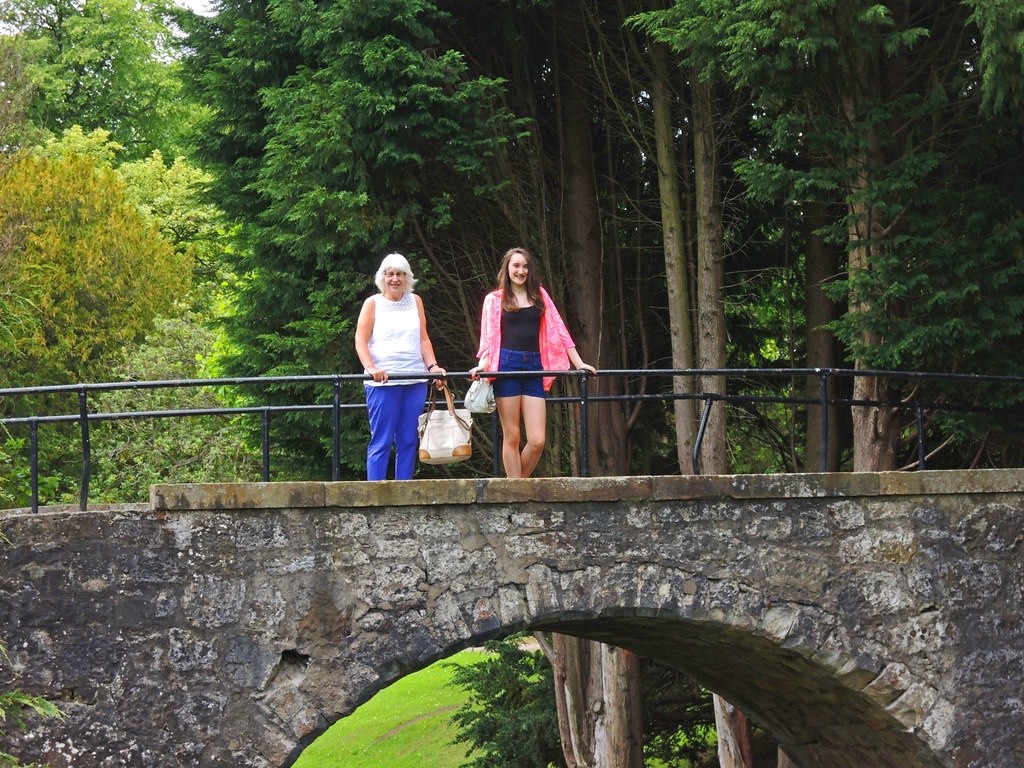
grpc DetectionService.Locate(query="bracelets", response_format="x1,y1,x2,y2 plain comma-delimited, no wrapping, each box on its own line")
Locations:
428,363,438,371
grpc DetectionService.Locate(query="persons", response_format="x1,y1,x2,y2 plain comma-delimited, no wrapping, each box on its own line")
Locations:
468,247,598,478
355,253,447,482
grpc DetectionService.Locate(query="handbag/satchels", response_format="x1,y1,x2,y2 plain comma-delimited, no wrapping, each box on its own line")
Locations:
417,380,473,464
464,378,497,414
